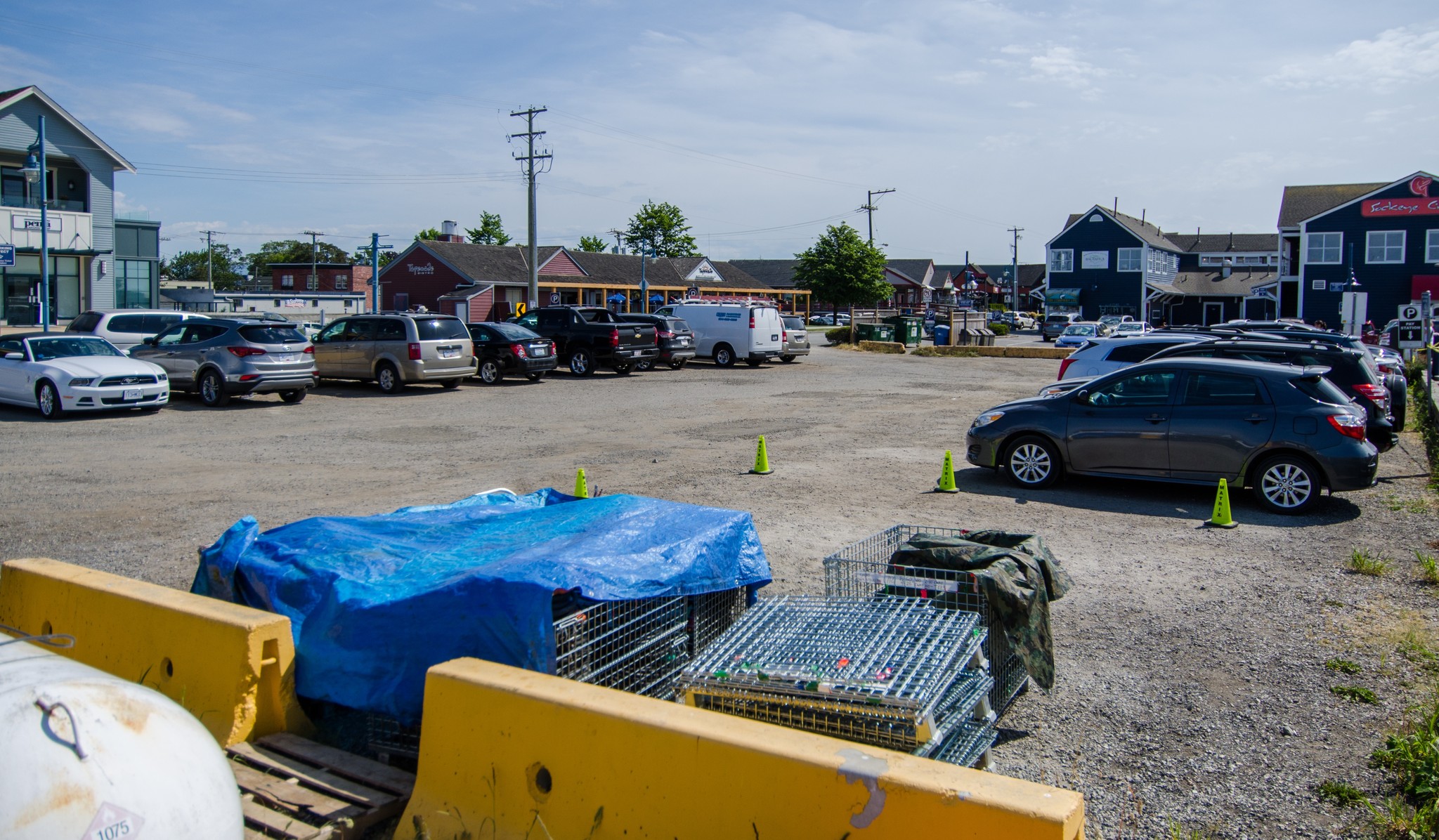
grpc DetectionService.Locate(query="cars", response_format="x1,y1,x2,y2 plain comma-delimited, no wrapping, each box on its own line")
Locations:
812,317,843,326
117,304,146,309
798,315,820,325
7,295,74,326
963,356,1379,514
986,313,1012,331
0,331,169,421
505,318,538,326
464,321,558,385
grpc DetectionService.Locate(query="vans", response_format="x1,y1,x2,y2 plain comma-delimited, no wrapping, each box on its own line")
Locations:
653,294,785,368
820,313,851,325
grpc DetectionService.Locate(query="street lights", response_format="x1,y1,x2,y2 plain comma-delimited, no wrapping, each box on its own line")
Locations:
963,251,979,347
1342,243,1362,293
641,238,660,314
1002,267,1017,311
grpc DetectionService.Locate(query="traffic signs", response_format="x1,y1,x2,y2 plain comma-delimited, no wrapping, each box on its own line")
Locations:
925,324,934,331
1397,304,1423,349
925,308,935,324
1421,292,1430,318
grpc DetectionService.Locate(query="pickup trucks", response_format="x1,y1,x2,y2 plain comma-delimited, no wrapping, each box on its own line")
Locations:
511,304,660,377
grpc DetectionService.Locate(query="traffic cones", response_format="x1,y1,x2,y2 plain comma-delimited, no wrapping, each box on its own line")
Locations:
574,468,588,499
748,435,774,475
1203,479,1239,528
934,450,960,494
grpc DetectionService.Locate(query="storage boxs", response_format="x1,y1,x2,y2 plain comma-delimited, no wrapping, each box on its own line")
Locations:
690,581,747,654
819,523,1044,731
550,590,688,705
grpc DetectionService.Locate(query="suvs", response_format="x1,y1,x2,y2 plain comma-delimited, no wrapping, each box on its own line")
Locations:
1043,313,1088,342
302,323,339,339
610,313,696,372
778,313,811,362
64,309,212,351
1096,315,1134,330
310,310,479,395
1003,311,1036,330
127,317,320,407
1038,320,1407,454
